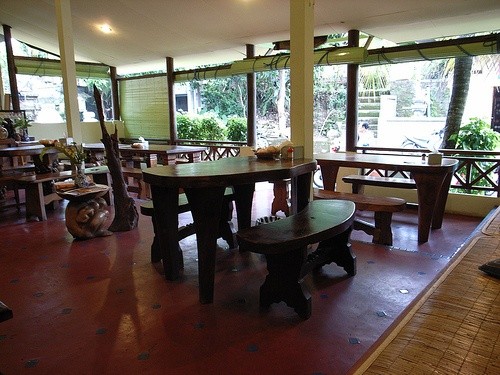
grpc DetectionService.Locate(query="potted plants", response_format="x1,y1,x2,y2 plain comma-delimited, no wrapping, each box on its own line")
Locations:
13,119,33,140
40,140,95,187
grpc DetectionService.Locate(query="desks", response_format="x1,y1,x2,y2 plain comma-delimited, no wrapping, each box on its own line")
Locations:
80,143,205,198
1,146,62,173
316,151,458,246
143,157,318,304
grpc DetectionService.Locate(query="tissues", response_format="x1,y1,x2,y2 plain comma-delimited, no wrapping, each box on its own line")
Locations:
59,138,73,147
281,135,304,159
138,136,148,149
428,144,443,165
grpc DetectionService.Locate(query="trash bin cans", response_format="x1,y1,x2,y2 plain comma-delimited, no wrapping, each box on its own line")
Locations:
255,215,283,225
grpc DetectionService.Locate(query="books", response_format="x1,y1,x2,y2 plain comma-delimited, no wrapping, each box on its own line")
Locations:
4,94,13,110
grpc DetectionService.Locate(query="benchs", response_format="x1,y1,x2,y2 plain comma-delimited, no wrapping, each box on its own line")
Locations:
237,199,356,320
0,159,199,236
314,188,406,246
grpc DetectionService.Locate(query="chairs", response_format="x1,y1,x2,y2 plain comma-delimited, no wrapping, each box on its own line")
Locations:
343,174,417,195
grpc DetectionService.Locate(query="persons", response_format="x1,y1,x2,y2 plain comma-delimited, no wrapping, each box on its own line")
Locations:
358,122,374,147
